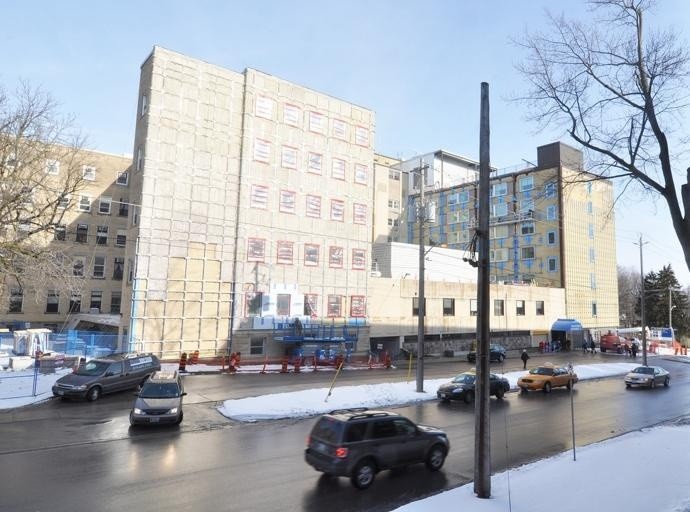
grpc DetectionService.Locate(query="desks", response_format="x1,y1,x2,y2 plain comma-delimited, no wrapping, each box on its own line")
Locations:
669,305,676,329
641,294,663,366
415,242,449,392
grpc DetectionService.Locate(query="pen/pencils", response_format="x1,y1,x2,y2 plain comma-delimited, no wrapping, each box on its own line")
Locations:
130,370,188,428
51,350,160,401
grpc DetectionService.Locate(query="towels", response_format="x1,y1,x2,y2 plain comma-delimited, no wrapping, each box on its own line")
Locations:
444,350,454,357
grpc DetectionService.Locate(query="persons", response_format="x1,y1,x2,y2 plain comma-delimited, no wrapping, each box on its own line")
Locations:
565,337,571,352
632,344,637,358
583,341,597,354
521,349,530,369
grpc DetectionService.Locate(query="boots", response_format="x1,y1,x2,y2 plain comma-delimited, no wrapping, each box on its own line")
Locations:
466,344,507,362
303,407,449,490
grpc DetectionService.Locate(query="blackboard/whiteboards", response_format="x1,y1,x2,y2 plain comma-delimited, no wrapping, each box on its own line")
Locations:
599,330,632,355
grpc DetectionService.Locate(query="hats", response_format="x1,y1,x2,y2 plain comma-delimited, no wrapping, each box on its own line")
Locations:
438,367,510,404
622,365,672,389
516,362,579,393
